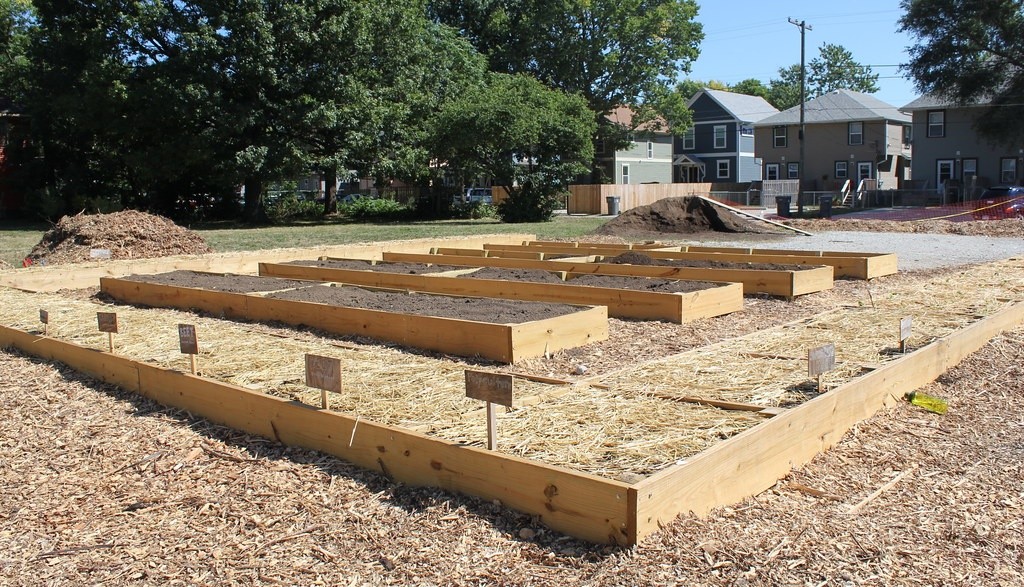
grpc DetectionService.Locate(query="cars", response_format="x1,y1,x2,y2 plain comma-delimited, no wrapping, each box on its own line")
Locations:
342,194,374,204
974,186,1024,219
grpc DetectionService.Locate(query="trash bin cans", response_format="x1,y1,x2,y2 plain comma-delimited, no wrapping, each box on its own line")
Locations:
775,195,791,218
606,196,621,215
819,195,832,217
748,189,761,205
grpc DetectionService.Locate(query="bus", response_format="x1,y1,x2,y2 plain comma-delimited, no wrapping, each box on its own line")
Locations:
453,188,492,206
268,190,325,207
